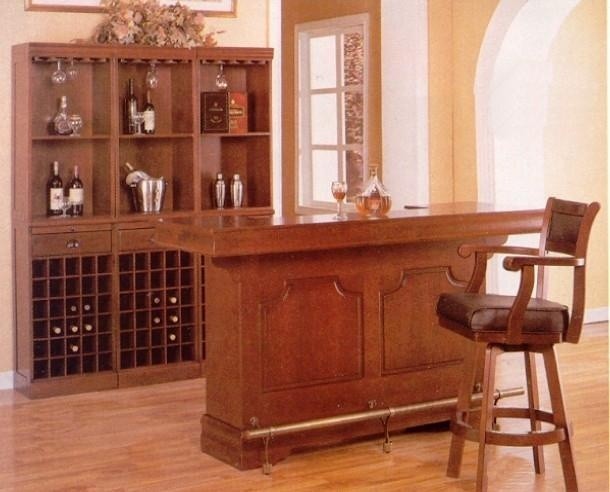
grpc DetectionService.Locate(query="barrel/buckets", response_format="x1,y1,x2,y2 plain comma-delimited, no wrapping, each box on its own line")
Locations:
131,177,166,215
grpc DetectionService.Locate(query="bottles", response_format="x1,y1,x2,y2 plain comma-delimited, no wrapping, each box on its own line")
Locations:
66,165,84,215
354,163,393,219
142,88,156,134
124,79,138,133
230,174,244,208
47,161,64,215
53,95,73,136
213,173,226,209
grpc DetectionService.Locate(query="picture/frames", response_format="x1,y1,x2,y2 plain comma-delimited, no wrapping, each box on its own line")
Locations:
24,1,238,18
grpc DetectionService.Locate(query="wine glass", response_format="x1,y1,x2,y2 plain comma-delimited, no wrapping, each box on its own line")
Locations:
330,180,350,219
145,61,162,88
216,62,229,90
51,58,67,84
67,115,83,137
133,111,145,135
67,57,81,81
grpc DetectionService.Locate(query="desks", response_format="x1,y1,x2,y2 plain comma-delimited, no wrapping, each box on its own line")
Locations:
147,200,546,470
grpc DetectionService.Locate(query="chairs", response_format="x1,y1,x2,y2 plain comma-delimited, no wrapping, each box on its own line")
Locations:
437,197,600,492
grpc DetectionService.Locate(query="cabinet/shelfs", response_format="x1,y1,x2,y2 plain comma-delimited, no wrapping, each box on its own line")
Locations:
13,42,114,224
195,47,275,222
15,225,117,400
119,223,207,388
113,44,195,222
